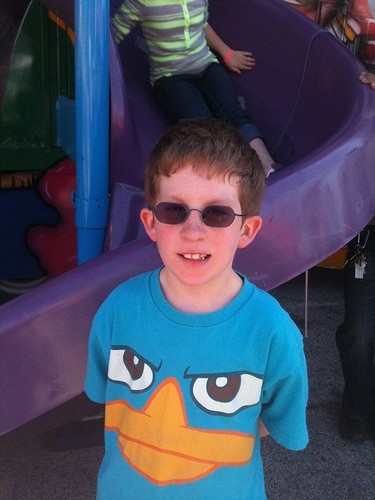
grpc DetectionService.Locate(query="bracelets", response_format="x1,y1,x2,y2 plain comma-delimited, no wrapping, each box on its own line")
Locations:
224,49,234,64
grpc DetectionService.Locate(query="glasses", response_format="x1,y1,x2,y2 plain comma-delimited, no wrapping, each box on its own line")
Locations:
147,203,251,228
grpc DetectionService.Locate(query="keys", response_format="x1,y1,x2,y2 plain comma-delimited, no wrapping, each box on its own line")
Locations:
343,229,371,278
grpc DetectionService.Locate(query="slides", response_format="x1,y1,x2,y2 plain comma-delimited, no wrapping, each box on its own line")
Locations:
0,0,375,435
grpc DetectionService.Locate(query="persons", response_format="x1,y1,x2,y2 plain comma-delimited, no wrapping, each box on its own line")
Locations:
84,117,308,500
110,0,284,178
336,72,375,441
